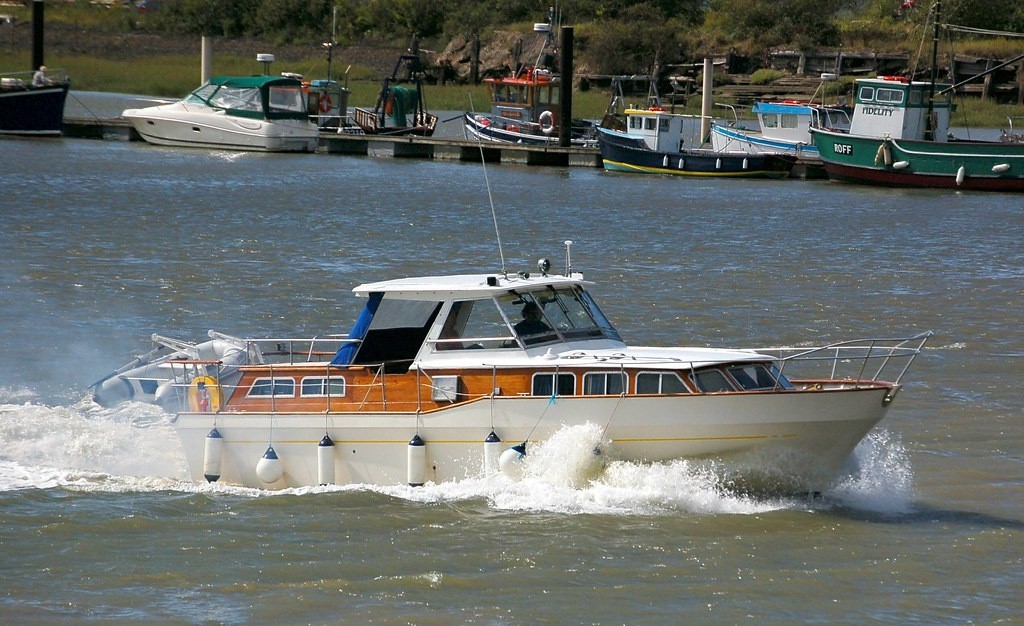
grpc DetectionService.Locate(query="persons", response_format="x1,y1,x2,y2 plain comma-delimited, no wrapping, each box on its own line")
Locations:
511,302,556,348
32,65,56,89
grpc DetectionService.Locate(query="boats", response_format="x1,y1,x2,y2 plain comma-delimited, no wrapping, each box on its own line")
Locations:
93,88,933,498
121,13,821,182
1,69,71,138
806,2,1024,192
